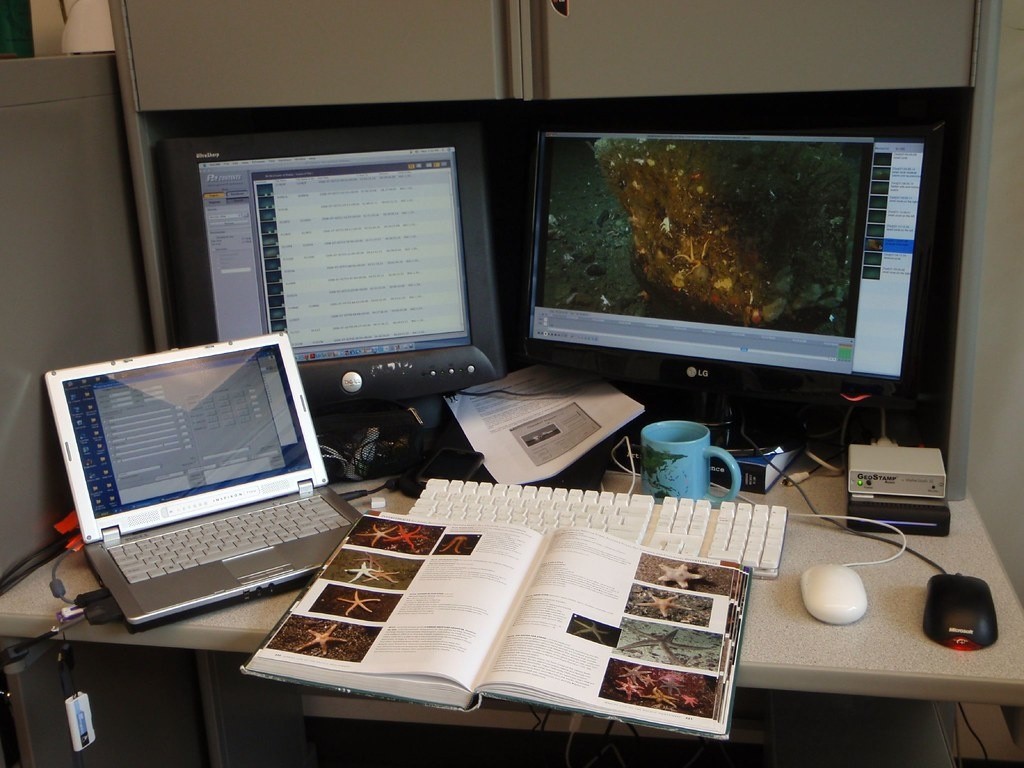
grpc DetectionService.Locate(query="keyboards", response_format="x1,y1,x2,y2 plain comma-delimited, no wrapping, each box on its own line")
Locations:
407,478,788,580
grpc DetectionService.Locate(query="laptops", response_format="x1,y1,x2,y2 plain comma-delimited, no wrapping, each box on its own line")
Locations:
43,330,364,634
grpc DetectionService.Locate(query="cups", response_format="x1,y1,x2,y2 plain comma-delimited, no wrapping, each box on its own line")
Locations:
640,420,741,507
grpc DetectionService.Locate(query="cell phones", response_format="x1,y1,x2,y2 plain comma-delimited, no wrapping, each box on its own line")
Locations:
417,448,484,483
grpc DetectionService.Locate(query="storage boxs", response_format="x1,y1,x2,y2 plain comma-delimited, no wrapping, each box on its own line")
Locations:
954,703,1024,768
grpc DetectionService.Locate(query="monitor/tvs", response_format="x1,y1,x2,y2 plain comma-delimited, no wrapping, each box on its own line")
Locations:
518,85,975,458
144,98,525,497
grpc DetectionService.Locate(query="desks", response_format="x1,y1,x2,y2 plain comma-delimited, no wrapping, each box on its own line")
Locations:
0,411,1024,768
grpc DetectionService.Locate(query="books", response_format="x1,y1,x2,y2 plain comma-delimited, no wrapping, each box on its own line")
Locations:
242,511,754,740
607,412,809,496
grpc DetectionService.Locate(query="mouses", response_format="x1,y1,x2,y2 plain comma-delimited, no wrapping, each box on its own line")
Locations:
921,572,998,651
800,563,867,624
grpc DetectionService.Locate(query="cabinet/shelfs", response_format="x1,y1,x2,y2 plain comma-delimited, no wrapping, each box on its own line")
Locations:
106,0,1024,498
